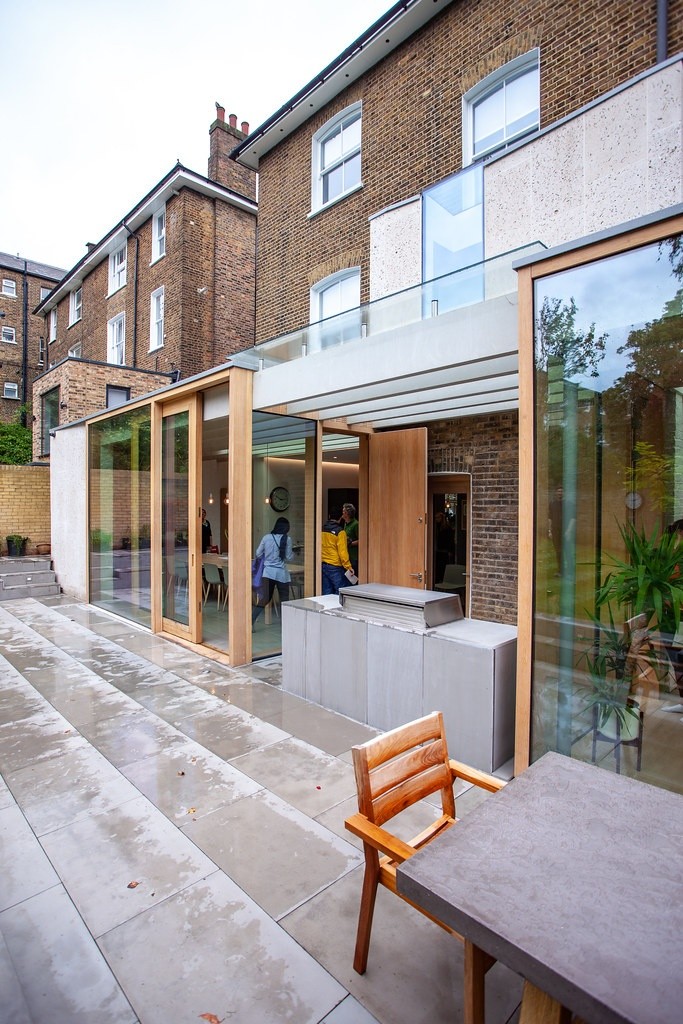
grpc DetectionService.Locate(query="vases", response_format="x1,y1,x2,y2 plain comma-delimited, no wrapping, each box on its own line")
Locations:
36,543,51,555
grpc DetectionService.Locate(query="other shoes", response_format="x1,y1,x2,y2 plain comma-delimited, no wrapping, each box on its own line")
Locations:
252,627,255,633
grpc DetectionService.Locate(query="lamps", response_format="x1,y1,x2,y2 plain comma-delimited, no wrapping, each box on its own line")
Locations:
33,415,36,421
60,401,67,409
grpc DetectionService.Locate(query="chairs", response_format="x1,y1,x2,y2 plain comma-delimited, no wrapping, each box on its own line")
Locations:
621,614,682,719
435,565,465,604
345,712,509,1024
167,560,304,622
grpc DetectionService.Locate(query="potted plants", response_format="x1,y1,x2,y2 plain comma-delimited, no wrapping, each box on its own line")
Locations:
6,535,28,556
122,525,149,550
574,515,683,742
90,529,112,553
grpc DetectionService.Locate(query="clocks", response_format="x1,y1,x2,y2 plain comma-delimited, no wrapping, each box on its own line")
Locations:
269,486,291,512
626,491,643,509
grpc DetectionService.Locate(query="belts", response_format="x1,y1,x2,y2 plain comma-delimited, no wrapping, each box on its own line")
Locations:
323,562,332,565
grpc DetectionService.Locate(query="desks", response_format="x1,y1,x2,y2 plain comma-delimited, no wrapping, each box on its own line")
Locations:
398,751,683,1024
199,554,303,625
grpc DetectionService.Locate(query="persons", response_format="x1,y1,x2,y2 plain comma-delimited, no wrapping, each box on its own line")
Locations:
434,513,452,568
321,503,360,594
252,517,300,633
202,510,212,554
654,519,683,723
